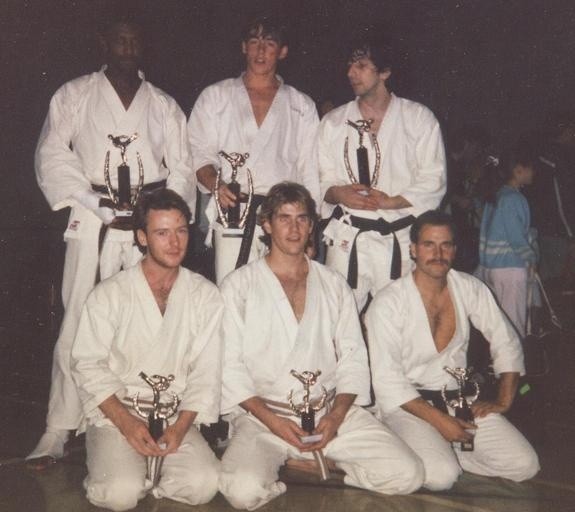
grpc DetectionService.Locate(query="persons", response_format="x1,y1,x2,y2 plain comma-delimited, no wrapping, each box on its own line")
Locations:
363,209,543,493
477,147,552,379
451,120,494,229
318,23,451,315
186,11,322,288
23,12,199,472
535,111,574,300
219,177,427,512
67,189,222,512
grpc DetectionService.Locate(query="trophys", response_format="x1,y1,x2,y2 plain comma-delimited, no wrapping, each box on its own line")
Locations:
289,368,328,443
343,117,381,197
440,365,481,434
132,370,179,451
213,151,253,238
103,131,144,229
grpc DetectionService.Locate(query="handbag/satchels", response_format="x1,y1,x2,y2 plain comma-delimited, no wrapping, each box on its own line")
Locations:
519,318,574,376
